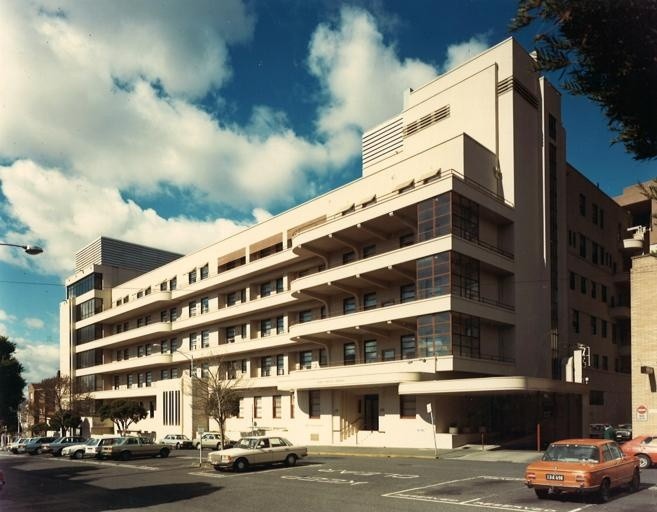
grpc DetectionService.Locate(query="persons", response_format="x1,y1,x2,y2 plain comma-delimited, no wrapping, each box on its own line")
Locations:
604,423,617,440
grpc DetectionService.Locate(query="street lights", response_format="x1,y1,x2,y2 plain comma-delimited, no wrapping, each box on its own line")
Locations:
0,241,43,257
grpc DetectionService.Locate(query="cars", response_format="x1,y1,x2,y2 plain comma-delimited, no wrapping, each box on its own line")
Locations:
525,439,641,504
85,434,121,457
40,436,85,457
26,437,56,455
612,424,632,440
207,435,309,474
589,423,611,438
192,431,238,451
100,437,172,461
60,436,94,460
158,434,192,450
16,439,34,454
5,437,24,455
619,435,657,470
